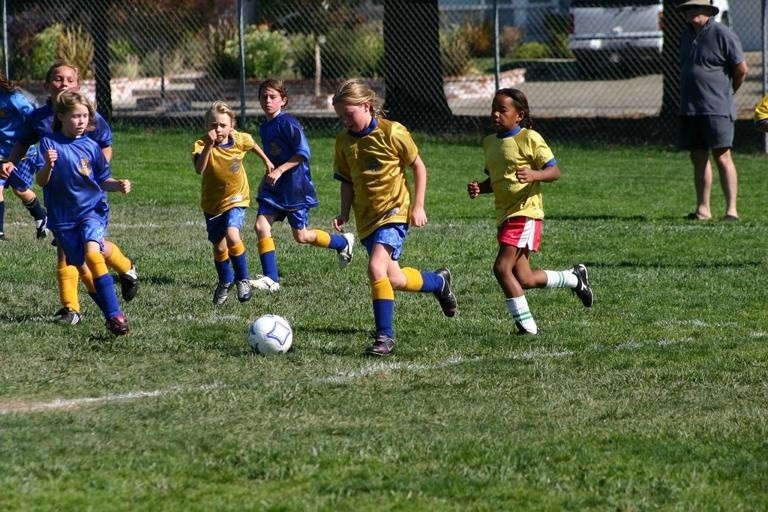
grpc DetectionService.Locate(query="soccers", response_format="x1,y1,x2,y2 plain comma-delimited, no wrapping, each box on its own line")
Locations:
249,312,292,356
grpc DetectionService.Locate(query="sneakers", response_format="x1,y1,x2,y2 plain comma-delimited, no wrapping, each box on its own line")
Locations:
105,315,130,336
35,208,49,240
366,334,396,357
435,268,457,317
338,232,355,269
54,306,81,326
569,263,593,307
120,256,138,302
212,273,280,307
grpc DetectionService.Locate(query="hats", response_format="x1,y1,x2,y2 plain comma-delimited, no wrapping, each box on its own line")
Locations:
675,0,718,16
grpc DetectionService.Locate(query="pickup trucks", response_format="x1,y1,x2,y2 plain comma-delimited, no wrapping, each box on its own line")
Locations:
568,1,734,71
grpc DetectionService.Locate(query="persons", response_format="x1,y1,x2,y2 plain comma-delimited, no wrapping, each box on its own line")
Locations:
191,98,276,306
248,77,356,294
467,87,594,336
673,0,750,223
0,60,139,325
331,76,460,356
0,72,50,240
34,86,131,336
